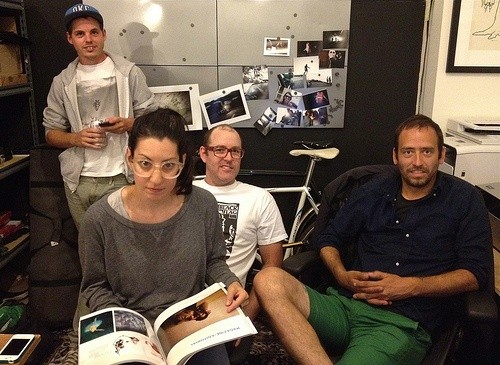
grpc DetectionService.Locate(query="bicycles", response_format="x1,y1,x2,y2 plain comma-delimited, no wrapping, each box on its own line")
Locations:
190,138,340,269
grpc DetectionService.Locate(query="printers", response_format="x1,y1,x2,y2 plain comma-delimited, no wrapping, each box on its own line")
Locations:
439,116,500,186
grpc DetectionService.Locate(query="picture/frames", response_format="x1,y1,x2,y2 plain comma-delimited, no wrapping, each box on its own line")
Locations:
446,0,500,74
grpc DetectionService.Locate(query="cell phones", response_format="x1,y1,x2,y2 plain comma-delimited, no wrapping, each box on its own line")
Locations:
98,121,114,127
0,333,35,364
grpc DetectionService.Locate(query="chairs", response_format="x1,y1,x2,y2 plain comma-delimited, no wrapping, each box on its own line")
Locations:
280,163,499,365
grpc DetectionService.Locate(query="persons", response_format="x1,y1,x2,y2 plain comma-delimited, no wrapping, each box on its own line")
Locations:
315,91,329,104
252,114,495,365
186,303,207,321
280,92,295,108
79,108,250,365
304,64,311,75
43,4,158,232
311,108,327,126
303,109,313,127
217,97,238,115
192,124,289,365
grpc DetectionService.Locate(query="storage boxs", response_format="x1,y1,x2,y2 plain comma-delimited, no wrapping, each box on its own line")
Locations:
0,15,28,90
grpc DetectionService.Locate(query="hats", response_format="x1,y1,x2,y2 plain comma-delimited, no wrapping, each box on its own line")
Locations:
63,4,103,31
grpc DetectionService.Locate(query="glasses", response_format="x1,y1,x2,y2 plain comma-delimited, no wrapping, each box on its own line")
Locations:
129,156,185,180
207,144,244,160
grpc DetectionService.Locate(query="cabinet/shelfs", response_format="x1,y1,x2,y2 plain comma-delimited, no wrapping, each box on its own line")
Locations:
0,0,44,277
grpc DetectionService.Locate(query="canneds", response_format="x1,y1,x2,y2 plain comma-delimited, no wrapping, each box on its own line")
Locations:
90,120,106,147
